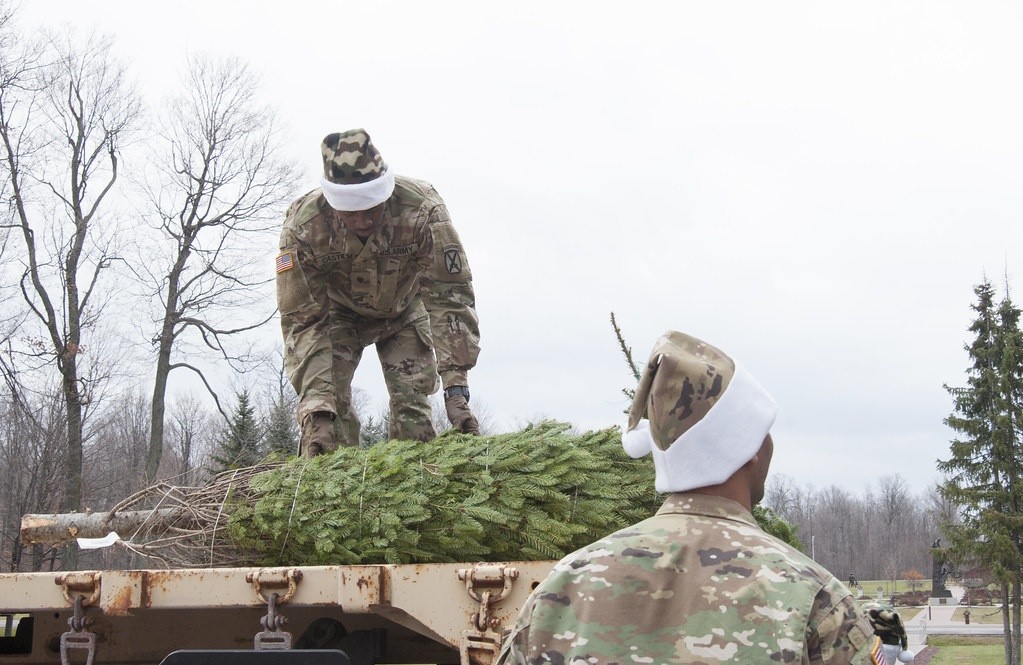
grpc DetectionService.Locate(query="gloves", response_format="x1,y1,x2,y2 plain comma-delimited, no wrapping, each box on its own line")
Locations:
302,413,335,461
446,395,481,436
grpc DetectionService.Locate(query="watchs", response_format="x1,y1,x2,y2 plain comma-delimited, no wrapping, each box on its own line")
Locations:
444,387,469,403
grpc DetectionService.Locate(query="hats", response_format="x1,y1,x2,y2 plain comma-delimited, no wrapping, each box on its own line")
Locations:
863,602,914,665
320,129,395,212
621,329,778,495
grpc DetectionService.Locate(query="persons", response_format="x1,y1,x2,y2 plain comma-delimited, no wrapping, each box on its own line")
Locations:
493,332,887,665
274,128,480,457
